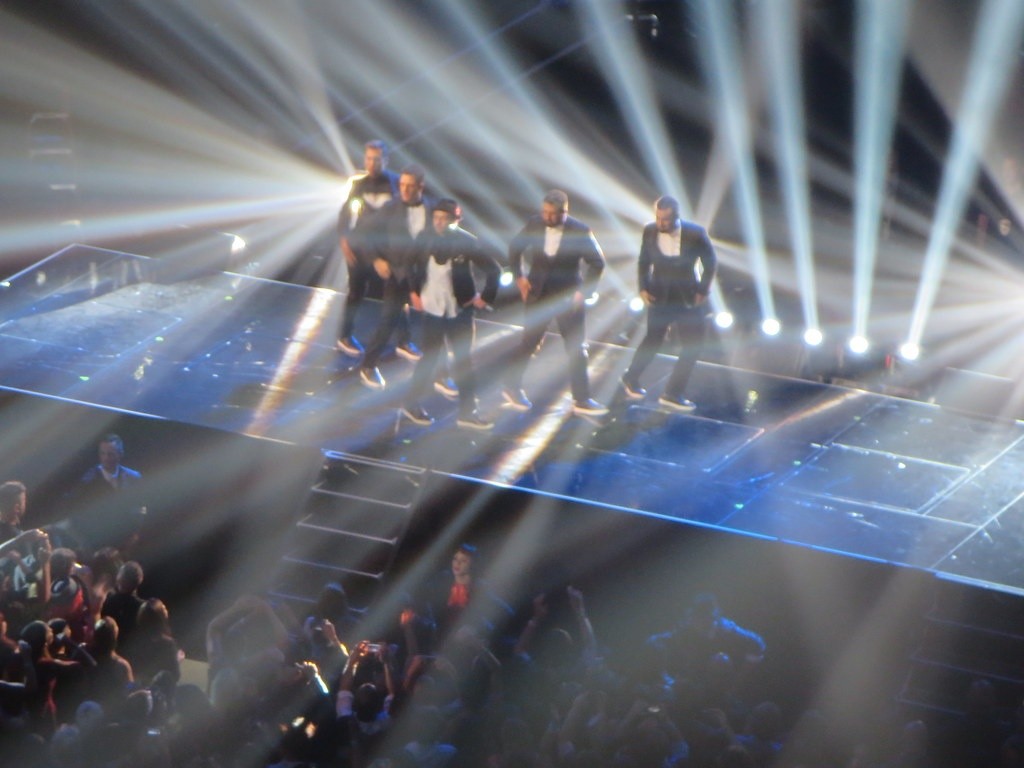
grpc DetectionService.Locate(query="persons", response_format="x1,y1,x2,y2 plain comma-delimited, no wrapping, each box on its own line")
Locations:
504,187,609,416
337,140,459,399
0,432,1024,768
621,195,716,411
398,198,503,429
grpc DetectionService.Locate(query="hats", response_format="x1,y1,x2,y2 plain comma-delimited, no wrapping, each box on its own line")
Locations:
429,198,463,222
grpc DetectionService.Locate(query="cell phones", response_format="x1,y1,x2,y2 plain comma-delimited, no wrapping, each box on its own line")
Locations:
366,643,381,652
311,620,324,630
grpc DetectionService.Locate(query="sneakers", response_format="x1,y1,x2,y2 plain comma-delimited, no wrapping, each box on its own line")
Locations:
619,369,648,399
574,398,610,416
501,387,532,409
434,377,459,396
399,396,435,426
360,366,385,387
659,392,696,411
337,334,365,355
396,342,423,360
457,411,495,430
